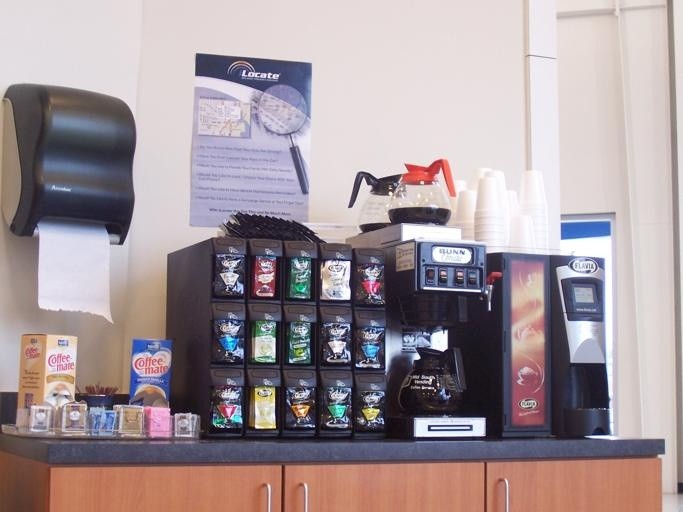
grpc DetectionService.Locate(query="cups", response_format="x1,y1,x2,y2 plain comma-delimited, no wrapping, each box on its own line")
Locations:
457,191,476,240
522,170,548,254
476,178,504,252
507,190,521,254
484,170,507,253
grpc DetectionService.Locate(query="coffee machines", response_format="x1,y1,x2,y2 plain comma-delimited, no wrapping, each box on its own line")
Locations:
344,223,502,442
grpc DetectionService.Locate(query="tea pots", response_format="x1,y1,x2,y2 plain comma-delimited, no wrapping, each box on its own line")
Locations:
348,171,401,233
388,159,456,225
398,346,467,417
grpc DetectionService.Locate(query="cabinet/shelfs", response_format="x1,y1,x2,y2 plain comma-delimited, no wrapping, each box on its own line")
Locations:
0,438,484,511
487,437,665,512
